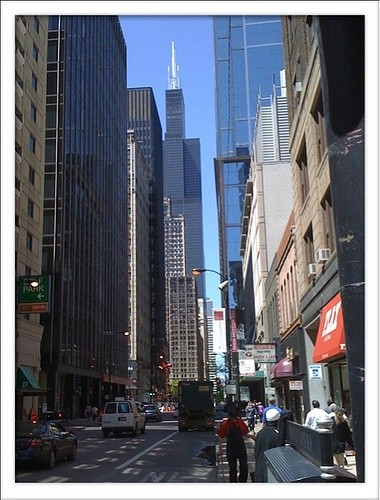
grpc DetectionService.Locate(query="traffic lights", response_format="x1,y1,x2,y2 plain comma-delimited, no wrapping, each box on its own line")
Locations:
149,392,156,396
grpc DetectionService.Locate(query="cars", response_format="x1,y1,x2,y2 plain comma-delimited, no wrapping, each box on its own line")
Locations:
173,406,178,418
143,404,162,422
15,419,78,471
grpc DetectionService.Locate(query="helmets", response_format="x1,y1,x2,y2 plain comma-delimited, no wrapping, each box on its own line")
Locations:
265,409,281,421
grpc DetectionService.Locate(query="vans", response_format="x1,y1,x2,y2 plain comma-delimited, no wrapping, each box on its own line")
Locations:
101,401,146,438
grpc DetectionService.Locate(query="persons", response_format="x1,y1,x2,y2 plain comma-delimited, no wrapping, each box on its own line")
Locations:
58,404,102,422
218,400,356,483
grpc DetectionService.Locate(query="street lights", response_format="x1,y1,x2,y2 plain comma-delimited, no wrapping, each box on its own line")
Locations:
108,331,131,403
15,281,40,419
191,268,232,402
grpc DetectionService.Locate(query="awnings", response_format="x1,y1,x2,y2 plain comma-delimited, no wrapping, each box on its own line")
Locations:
313,291,346,363
270,355,293,380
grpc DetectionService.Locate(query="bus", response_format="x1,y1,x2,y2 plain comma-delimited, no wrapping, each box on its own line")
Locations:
177,381,217,432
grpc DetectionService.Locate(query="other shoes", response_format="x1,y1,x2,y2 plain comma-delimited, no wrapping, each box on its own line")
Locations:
344,457,347,465
